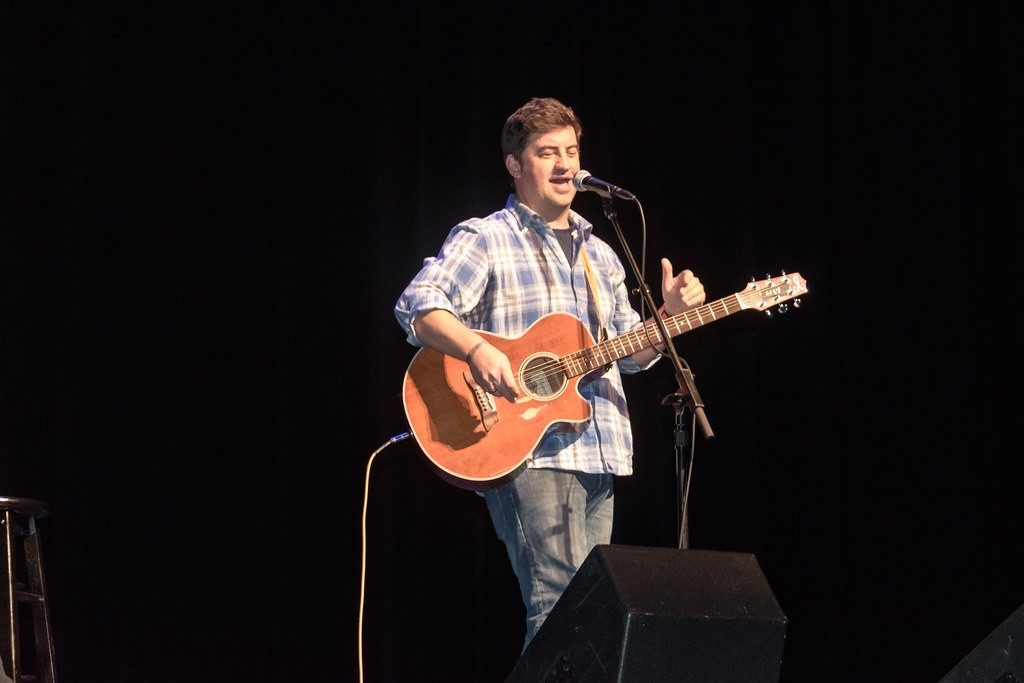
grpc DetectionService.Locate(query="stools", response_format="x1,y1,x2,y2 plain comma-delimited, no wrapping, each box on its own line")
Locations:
0,495,59,683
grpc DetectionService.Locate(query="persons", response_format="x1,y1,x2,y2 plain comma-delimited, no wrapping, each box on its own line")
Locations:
393,98,705,656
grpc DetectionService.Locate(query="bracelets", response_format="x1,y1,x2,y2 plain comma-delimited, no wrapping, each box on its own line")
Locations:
465,340,487,362
661,306,671,318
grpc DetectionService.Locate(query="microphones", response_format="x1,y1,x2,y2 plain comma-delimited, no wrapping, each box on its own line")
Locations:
572,168,635,202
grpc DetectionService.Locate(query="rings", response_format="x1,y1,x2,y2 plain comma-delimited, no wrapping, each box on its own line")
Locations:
491,389,497,393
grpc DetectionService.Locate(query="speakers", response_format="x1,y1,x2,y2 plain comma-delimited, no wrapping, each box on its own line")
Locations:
938,603,1024,682
504,543,788,683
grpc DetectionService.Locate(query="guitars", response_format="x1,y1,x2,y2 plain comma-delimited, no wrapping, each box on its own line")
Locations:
405,269,811,485
